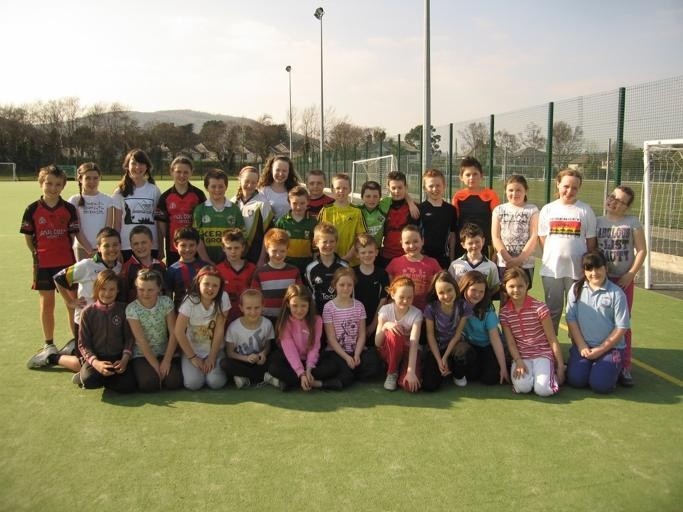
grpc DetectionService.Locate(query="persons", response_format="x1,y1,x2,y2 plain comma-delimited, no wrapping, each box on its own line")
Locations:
451,157,502,264
72,270,134,388
318,173,368,261
109,148,165,261
67,162,114,261
358,181,420,249
307,168,335,220
379,170,416,267
374,276,423,393
123,225,166,281
252,227,304,323
304,222,355,314
230,166,273,257
174,265,232,390
447,223,501,300
491,175,540,292
422,270,474,392
215,230,257,322
323,267,377,387
157,156,207,265
538,169,597,337
385,224,442,309
565,250,631,393
20,164,81,356
125,268,182,393
191,168,242,264
26,227,122,372
220,289,280,390
499,266,565,397
275,185,317,268
167,225,212,292
458,271,511,385
267,284,343,392
418,169,457,270
352,233,388,347
258,154,299,217
594,187,647,386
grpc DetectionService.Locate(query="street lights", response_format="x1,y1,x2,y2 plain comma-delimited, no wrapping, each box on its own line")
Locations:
314,6,325,170
285,65,293,159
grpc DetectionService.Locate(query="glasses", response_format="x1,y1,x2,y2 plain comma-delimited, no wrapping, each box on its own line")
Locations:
607,194,625,206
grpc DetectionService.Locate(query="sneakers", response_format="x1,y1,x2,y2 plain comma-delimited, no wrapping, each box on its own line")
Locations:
234,376,250,389
320,378,343,391
383,373,398,390
27,344,58,369
452,376,467,386
71,372,82,384
619,368,634,386
263,372,280,388
58,338,75,355
